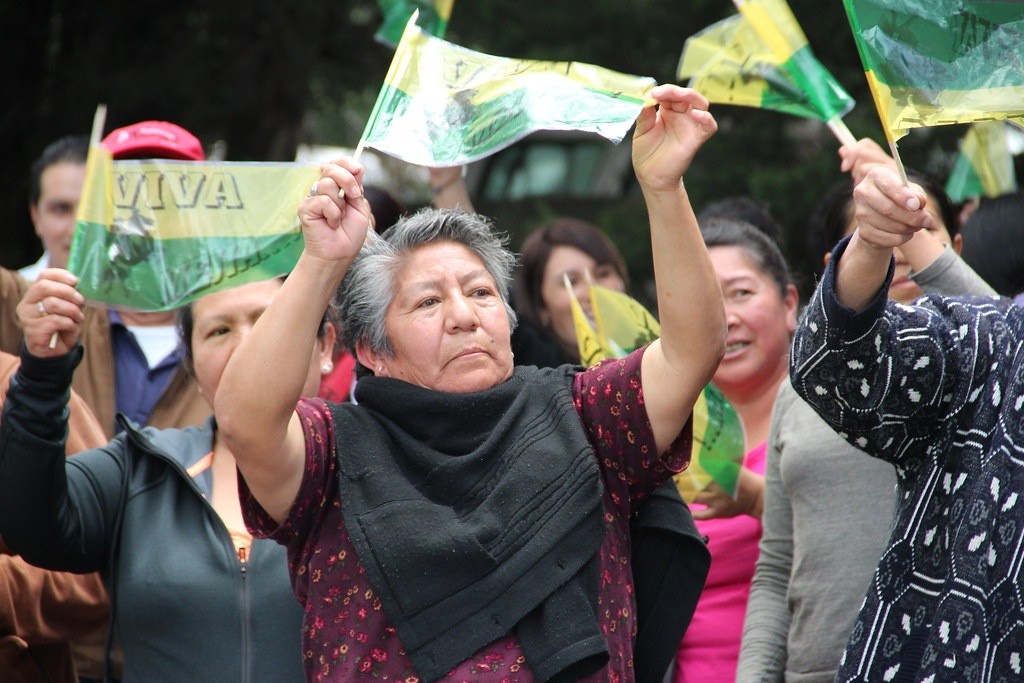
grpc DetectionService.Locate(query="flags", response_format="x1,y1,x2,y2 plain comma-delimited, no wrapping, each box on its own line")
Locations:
941,116,1019,205
370,0,457,53
360,22,660,169
674,1,857,123
843,0,1024,151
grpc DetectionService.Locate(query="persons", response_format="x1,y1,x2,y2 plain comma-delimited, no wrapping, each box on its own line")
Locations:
663,198,798,683
789,137,1024,683
213,80,729,683
733,168,964,683
0,120,376,683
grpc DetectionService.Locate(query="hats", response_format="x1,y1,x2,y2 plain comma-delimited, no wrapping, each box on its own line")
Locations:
101,120,205,162
